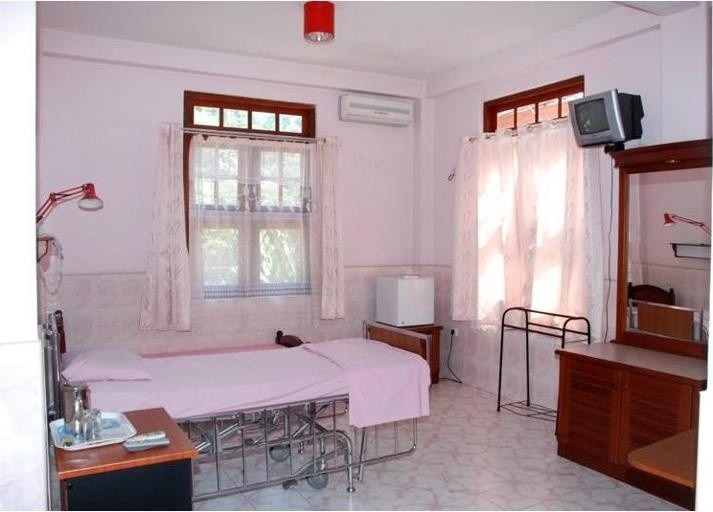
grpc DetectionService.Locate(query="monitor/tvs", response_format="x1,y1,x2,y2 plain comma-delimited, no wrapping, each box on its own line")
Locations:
568,87,645,147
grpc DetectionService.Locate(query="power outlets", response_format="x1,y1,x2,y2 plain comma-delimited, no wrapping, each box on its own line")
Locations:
450,328,458,336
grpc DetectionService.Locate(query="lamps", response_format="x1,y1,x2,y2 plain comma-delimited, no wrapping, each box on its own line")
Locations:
304,1,335,42
663,212,711,237
36,183,104,222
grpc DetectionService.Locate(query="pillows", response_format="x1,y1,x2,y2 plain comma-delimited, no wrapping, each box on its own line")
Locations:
61,348,153,382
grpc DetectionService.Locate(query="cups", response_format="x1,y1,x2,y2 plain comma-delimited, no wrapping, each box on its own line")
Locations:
62,384,104,442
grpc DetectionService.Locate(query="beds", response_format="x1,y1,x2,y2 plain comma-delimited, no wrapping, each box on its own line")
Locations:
627,281,708,344
44,311,431,502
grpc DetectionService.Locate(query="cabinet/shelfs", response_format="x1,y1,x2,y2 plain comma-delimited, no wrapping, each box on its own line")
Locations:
554,349,707,511
401,325,443,383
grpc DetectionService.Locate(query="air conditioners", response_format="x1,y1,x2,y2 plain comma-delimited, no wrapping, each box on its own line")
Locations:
338,95,414,127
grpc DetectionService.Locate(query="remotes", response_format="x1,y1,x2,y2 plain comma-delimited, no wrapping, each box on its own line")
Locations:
126,430,167,441
122,437,172,452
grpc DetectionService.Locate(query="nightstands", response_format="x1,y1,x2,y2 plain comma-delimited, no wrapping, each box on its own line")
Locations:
53,407,199,511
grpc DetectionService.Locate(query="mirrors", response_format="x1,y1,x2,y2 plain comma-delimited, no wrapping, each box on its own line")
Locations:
610,138,712,362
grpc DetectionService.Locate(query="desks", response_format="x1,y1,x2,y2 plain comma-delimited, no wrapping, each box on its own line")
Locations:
626,428,698,489
